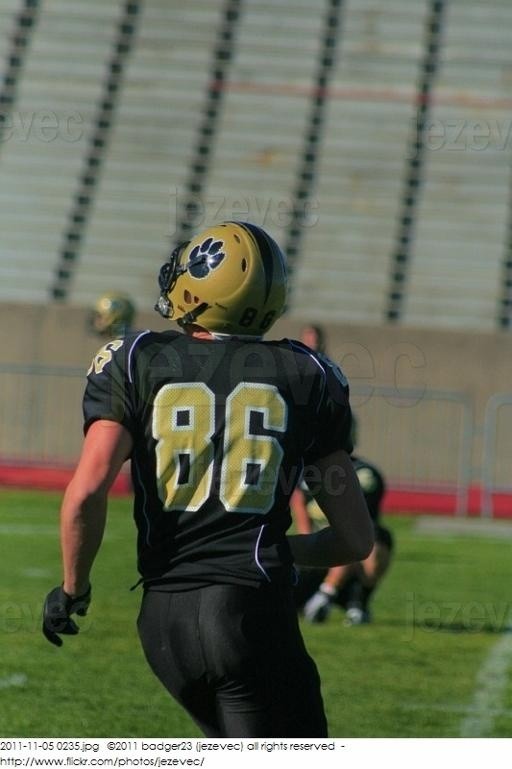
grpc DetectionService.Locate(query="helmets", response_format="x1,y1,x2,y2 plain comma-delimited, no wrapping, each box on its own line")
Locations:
90,294,132,333
162,219,289,339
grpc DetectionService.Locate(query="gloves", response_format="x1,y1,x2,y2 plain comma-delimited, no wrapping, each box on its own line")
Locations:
41,581,92,647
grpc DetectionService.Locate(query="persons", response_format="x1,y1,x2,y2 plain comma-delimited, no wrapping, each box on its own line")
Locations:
297,323,325,352
81,289,138,340
40,219,375,739
292,455,395,629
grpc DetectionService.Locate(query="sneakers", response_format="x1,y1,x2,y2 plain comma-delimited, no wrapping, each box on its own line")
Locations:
345,586,369,624
304,584,337,622
